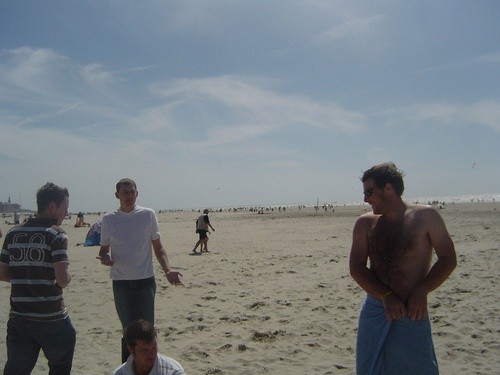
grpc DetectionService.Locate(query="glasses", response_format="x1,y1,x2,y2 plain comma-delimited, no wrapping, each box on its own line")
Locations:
364,182,386,196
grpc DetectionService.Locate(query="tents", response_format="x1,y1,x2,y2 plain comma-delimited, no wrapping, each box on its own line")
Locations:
82,218,103,245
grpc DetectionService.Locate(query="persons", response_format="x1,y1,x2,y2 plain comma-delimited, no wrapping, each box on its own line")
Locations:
1,182,77,375
14,210,21,224
348,161,457,375
98,177,191,363
76,211,91,227
111,318,186,375
193,209,215,254
